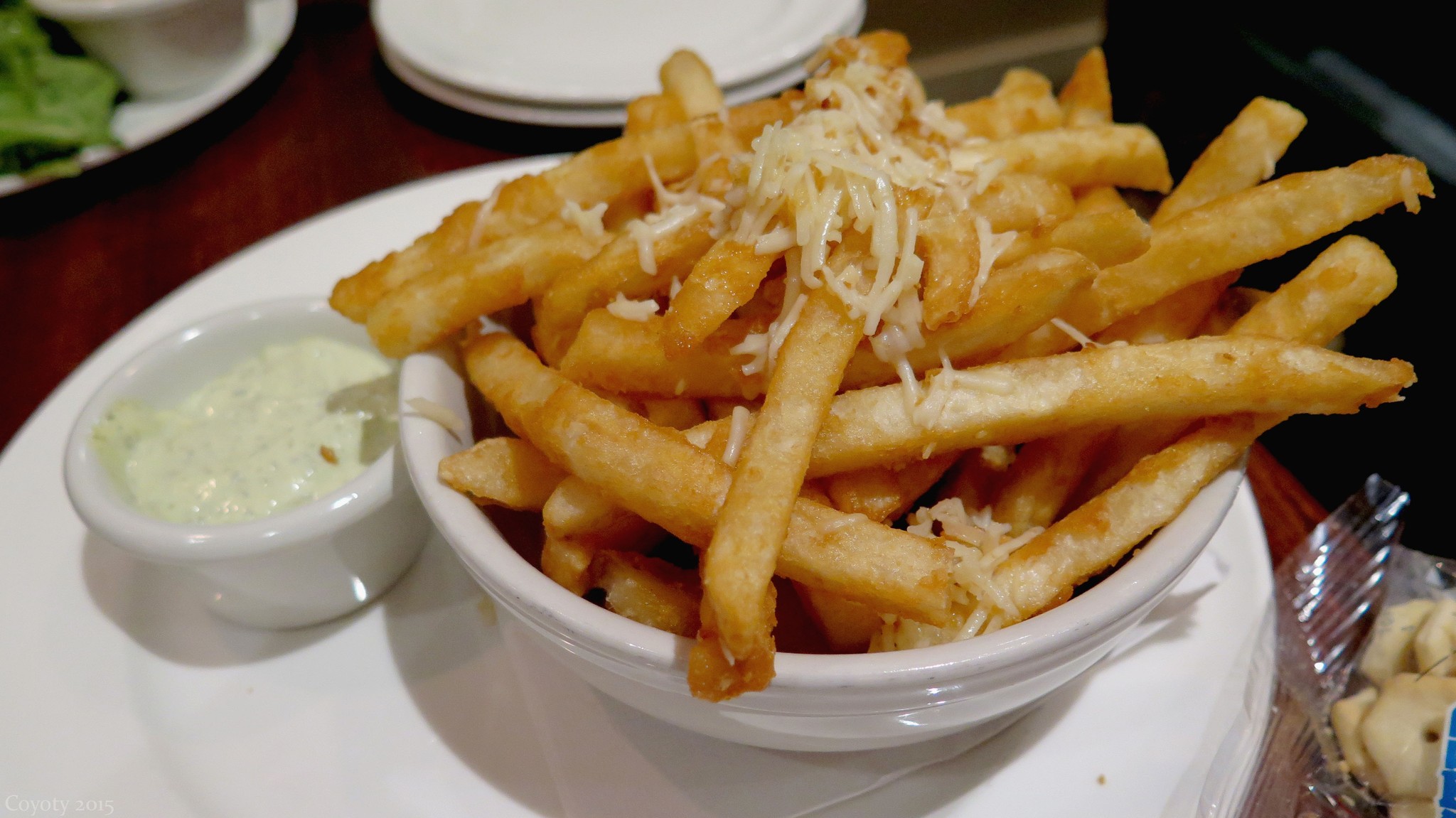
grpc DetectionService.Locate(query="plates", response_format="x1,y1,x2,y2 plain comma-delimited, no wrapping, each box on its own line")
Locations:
0,0,298,199
1,147,1275,818
370,0,868,127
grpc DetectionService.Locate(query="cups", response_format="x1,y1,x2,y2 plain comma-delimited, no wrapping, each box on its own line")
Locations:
63,299,431,630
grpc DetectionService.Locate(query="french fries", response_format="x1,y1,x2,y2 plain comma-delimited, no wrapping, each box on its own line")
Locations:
329,36,1432,700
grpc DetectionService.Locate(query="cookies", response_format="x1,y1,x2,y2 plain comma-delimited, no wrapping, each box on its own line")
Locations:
1329,593,1456,818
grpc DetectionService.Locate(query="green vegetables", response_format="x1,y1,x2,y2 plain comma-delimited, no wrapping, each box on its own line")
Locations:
0,0,139,184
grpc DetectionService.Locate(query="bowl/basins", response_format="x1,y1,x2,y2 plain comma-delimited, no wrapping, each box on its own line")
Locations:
398,333,1248,749
